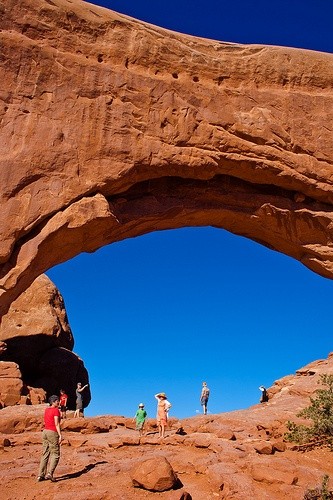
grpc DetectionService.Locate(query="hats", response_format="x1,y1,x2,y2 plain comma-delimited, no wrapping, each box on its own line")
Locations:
138,403,144,408
202,382,207,386
157,393,167,399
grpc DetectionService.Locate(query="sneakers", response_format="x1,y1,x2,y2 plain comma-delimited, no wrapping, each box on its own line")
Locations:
37,476,44,482
45,474,57,482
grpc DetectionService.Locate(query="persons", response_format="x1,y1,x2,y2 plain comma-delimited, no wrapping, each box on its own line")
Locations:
132,404,147,437
199,382,210,415
259,386,268,403
154,393,172,437
74,383,87,418
36,395,62,481
59,390,67,419
